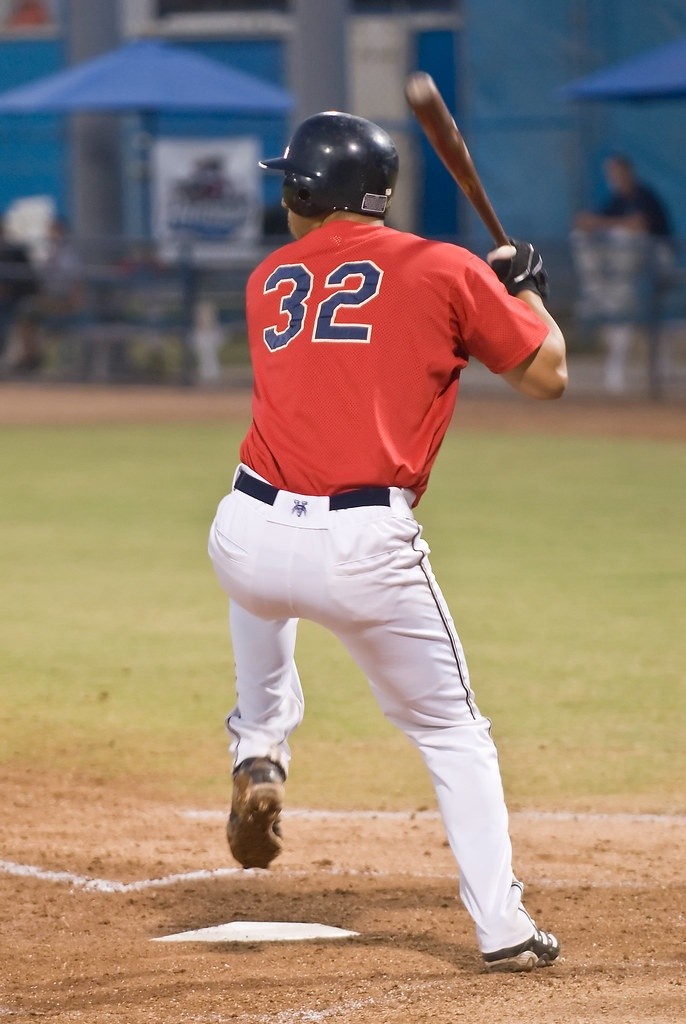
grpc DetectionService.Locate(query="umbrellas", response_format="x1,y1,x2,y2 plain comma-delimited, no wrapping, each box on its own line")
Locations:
559,34,686,103
0,40,295,261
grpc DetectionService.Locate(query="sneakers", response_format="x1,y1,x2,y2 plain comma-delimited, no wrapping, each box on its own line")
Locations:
226,757,283,869
484,930,560,972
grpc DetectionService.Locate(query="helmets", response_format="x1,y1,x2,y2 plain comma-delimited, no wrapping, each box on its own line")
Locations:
259,111,400,219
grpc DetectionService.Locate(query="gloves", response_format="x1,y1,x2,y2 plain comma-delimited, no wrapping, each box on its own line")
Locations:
487,237,550,300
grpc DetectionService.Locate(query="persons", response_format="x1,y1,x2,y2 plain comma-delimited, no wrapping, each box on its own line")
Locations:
574,152,675,398
0,211,83,372
206,108,567,972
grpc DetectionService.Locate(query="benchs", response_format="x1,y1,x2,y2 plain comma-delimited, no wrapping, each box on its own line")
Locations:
594,236,686,398
25,236,197,387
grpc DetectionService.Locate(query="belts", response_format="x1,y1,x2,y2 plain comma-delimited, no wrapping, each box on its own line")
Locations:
234,472,390,510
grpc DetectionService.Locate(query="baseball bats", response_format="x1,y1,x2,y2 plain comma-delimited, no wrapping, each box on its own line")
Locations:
402,70,513,250
